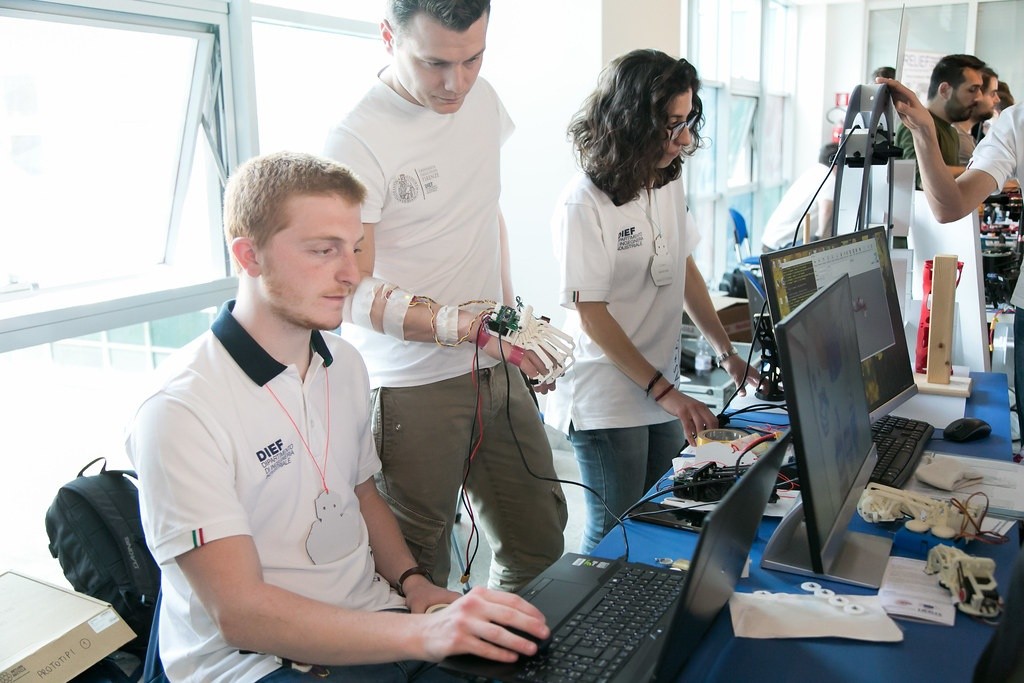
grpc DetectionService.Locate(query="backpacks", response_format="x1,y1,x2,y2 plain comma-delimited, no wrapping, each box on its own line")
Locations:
45,457,163,658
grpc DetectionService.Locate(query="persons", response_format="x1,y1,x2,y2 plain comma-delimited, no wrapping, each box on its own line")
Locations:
762,142,838,253
318,0,568,593
124,150,550,683
876,76,1024,448
895,55,985,191
559,49,770,556
951,68,1001,166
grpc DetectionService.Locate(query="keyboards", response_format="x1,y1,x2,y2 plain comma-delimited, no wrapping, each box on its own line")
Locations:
862,413,936,490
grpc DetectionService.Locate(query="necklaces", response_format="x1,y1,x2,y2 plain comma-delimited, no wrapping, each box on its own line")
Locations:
265,363,360,565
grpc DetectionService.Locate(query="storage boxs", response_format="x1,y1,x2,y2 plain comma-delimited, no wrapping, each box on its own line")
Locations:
0,571,138,683
682,296,752,344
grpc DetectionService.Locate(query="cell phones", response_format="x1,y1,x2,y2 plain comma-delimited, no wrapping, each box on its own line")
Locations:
628,501,707,532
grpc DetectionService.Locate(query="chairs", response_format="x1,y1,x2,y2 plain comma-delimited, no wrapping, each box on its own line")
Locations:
729,209,766,302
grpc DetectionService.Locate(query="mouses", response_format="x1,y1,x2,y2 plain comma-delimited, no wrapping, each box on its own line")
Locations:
943,418,991,442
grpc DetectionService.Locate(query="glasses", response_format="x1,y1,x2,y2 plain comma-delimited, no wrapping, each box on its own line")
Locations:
667,111,700,140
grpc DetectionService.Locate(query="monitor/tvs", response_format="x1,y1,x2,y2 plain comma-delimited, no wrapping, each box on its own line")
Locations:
760,222,919,587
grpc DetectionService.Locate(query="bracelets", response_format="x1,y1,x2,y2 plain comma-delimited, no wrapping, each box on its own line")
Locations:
396,567,434,598
655,384,674,401
645,370,662,397
715,346,738,363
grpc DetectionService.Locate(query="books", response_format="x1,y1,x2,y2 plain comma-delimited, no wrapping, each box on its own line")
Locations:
900,451,1024,519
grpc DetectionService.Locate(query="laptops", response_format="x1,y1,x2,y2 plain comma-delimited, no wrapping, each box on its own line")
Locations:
436,428,797,683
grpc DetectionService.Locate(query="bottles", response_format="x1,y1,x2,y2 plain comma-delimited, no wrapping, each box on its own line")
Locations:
694,334,713,376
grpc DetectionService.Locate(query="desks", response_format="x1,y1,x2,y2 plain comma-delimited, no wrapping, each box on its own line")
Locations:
592,373,1021,683
